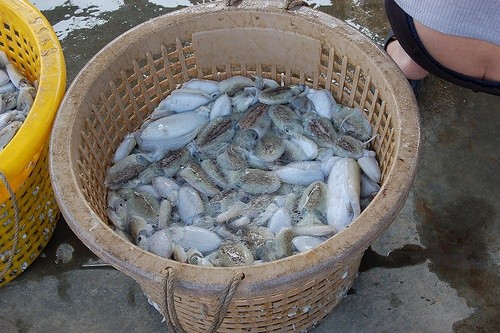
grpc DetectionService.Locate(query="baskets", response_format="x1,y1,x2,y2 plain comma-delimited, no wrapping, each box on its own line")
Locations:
50,0,420,333
0,0,66,290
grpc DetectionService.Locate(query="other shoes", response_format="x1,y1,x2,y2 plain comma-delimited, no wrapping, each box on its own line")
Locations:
383,26,423,95
